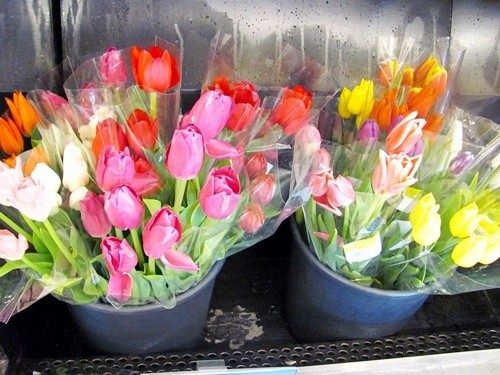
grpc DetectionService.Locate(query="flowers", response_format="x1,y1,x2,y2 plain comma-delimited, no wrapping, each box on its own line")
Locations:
0,38,339,324
299,38,500,297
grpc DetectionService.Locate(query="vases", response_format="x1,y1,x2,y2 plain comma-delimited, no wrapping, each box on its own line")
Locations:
72,260,223,354
292,214,431,342
